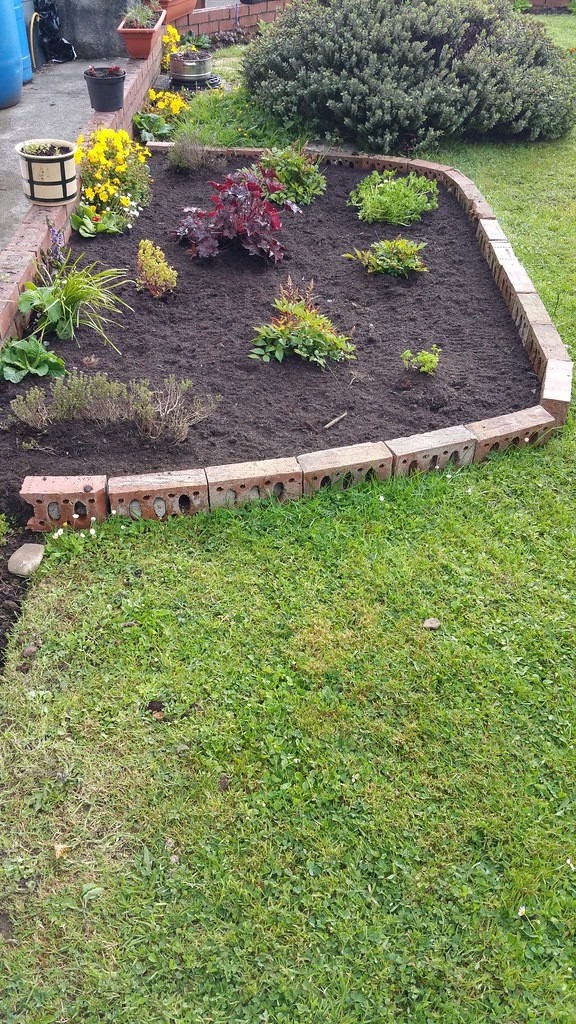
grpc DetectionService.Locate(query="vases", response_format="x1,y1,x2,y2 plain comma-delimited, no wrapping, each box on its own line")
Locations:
84,68,127,112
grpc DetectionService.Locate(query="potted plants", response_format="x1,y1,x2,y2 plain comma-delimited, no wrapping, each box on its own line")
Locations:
15,139,80,206
115,1,168,59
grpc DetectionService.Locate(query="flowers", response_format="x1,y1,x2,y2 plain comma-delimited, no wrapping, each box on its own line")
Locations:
85,65,120,77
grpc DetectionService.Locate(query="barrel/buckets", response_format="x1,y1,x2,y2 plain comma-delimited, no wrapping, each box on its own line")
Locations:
0,0,23,108
13,0,32,85
